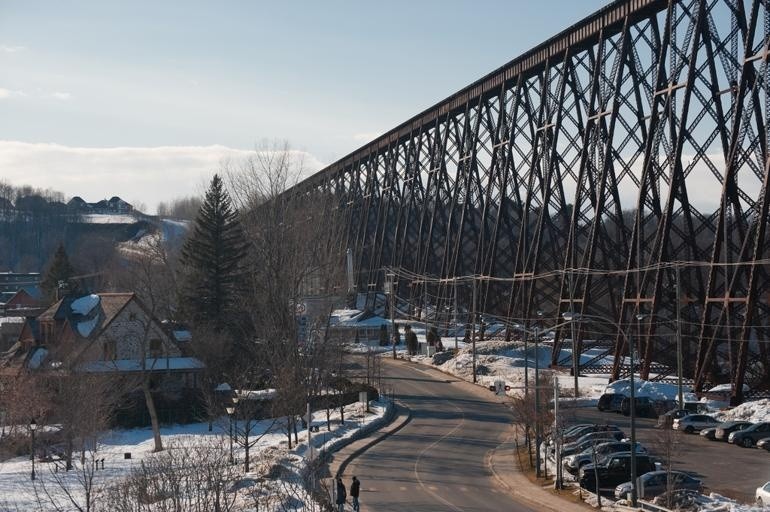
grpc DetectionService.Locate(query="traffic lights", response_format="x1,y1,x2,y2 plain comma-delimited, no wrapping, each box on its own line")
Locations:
503,386,511,391
490,386,496,392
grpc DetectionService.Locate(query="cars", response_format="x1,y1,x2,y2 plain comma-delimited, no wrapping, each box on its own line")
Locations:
755,477,770,512
597,393,770,452
547,424,702,501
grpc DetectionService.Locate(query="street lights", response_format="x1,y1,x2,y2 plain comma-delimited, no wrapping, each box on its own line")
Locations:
224,394,238,464
509,309,683,504
28,418,39,481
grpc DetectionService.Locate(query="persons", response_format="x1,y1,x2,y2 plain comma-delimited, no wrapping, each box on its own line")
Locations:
336,479,347,512
349,475,360,512
